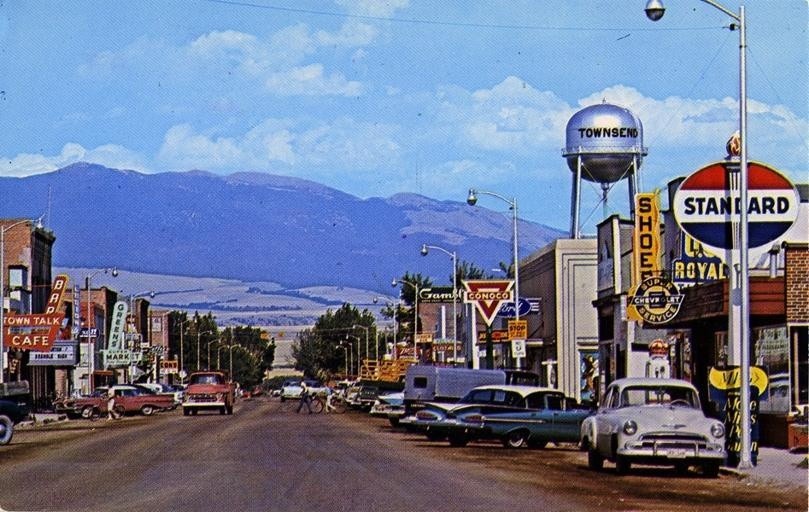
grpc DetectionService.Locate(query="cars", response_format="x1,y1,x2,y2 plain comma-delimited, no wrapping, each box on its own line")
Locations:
0,378,190,447
579,374,730,480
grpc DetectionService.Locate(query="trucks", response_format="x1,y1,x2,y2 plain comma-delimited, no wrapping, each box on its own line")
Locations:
179,372,236,417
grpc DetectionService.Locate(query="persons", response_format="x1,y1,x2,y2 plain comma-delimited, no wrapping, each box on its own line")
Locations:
320,381,336,414
294,378,313,414
103,381,117,421
234,380,243,405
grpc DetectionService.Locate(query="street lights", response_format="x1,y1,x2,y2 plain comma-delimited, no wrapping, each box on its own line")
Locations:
642,1,758,473
333,186,523,377
86,264,118,395
130,288,157,385
0,211,47,385
176,315,250,382
